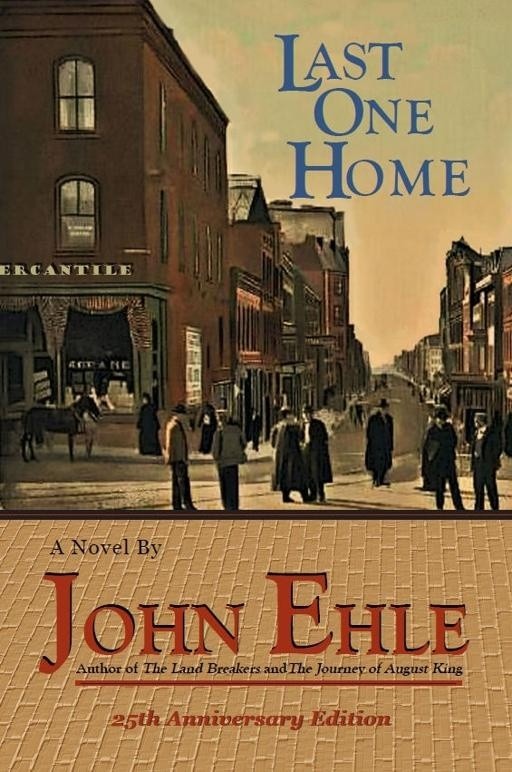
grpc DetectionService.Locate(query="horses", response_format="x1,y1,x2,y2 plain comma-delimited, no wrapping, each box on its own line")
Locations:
21,392,100,463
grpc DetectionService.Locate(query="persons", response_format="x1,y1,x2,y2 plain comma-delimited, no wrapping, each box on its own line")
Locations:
196,399,218,451
424,408,466,512
365,398,393,487
469,411,502,512
214,409,246,512
270,405,311,503
342,395,370,429
136,391,162,456
251,407,262,452
417,414,437,490
164,405,197,511
297,402,332,504
